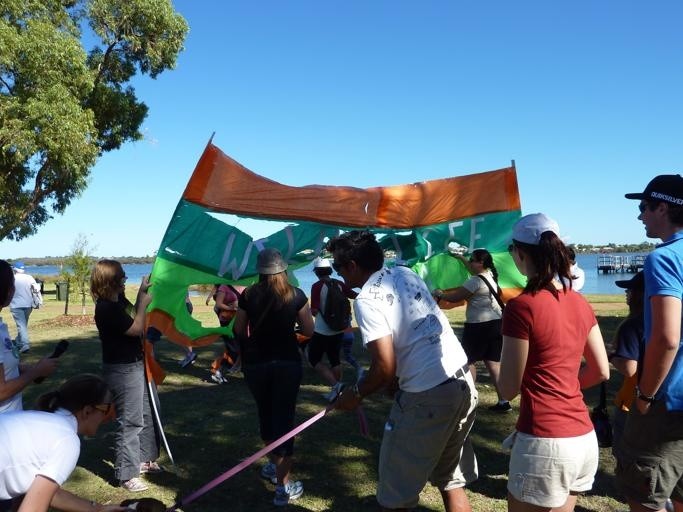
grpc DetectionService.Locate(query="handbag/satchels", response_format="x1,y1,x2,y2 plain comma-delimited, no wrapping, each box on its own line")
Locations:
29,282,42,309
588,406,613,448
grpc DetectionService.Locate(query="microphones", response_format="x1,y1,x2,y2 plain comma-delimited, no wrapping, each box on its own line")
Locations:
34,340,69,384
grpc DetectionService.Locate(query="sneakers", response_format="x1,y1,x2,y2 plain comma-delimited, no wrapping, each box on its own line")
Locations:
17,345,29,353
355,366,365,385
321,381,345,403
176,351,197,367
211,369,228,385
138,461,168,475
118,478,148,492
487,401,512,412
272,480,304,506
262,460,277,483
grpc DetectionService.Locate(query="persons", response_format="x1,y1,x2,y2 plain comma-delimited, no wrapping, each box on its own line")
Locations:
0,374,136,512
146,282,250,386
0,258,60,413
619,173,682,511
7,259,42,354
553,246,584,292
494,210,610,510
340,299,363,381
231,246,314,505
322,225,479,511
432,248,512,414
306,257,359,403
87,259,165,493
611,270,645,506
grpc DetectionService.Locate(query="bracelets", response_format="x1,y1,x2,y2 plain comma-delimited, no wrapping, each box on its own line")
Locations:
637,391,655,404
436,292,444,298
352,384,362,403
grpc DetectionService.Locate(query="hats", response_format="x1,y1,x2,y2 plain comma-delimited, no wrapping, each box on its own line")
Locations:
615,269,644,289
254,248,288,275
513,212,560,246
312,257,330,268
624,174,683,208
14,261,24,270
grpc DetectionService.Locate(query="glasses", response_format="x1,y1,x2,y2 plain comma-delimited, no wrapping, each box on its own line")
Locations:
638,202,650,213
507,243,517,255
120,271,126,279
331,262,344,272
92,402,112,416
469,258,479,263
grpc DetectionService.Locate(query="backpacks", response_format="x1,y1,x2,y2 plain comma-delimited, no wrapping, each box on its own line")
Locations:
316,276,351,331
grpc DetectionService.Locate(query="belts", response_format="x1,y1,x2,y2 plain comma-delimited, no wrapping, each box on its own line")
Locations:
435,363,469,387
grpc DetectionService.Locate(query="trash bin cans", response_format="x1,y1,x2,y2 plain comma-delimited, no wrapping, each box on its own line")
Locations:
55,282,67,301
